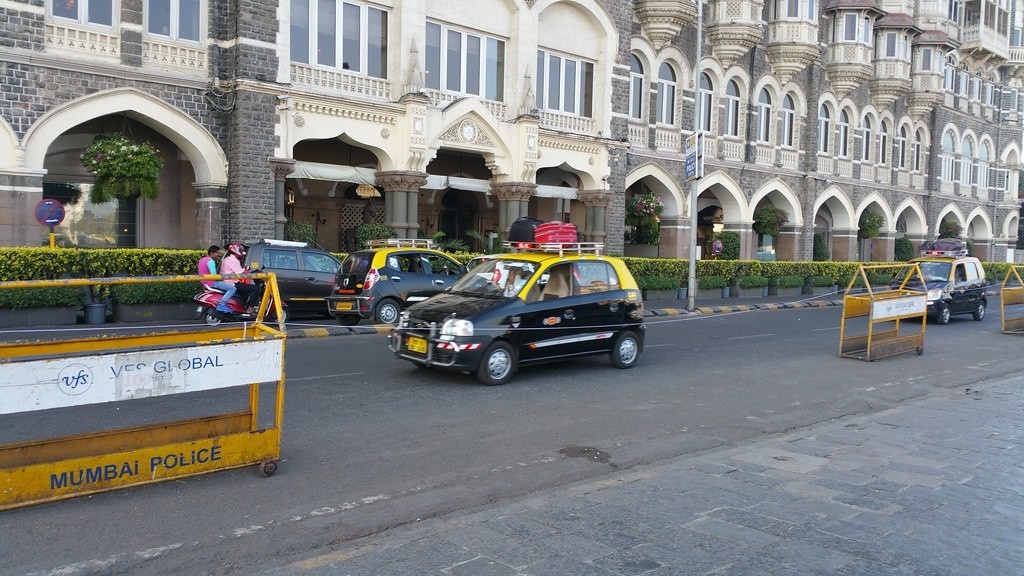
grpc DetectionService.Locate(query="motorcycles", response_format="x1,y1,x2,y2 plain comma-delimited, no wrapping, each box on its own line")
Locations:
192,262,290,329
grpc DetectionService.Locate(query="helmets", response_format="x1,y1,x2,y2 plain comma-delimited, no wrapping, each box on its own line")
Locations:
229,243,246,260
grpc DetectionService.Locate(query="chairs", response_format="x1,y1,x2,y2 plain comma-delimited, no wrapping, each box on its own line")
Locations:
521,271,543,303
407,261,421,272
538,267,569,301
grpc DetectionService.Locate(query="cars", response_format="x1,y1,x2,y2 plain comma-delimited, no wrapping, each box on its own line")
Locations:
386,243,646,386
886,250,988,326
324,237,503,326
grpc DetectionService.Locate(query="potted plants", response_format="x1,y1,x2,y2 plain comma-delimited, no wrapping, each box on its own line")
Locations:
634,275,680,300
696,275,726,299
94,281,203,323
866,274,891,291
769,276,805,295
838,275,864,294
0,285,89,329
803,276,836,294
729,276,769,298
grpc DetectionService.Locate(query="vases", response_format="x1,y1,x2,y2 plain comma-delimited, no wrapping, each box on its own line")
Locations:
116,181,140,200
83,302,108,323
833,285,838,295
722,287,730,297
762,286,769,296
677,287,687,299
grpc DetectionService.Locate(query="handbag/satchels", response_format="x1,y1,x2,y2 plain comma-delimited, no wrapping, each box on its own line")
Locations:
508,217,543,248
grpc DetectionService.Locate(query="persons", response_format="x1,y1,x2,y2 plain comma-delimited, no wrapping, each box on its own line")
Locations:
507,266,525,284
220,243,260,317
197,245,237,313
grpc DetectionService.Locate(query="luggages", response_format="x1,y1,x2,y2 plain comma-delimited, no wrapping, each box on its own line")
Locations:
533,220,577,248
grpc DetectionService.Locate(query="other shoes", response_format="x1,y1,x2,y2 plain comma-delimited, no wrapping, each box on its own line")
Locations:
216,304,233,313
247,311,257,316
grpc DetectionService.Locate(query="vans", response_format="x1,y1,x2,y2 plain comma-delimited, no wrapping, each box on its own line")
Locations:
214,237,344,324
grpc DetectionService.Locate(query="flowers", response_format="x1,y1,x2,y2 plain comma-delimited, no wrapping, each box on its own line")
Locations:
79,131,164,205
753,205,790,235
625,191,664,226
859,212,885,239
